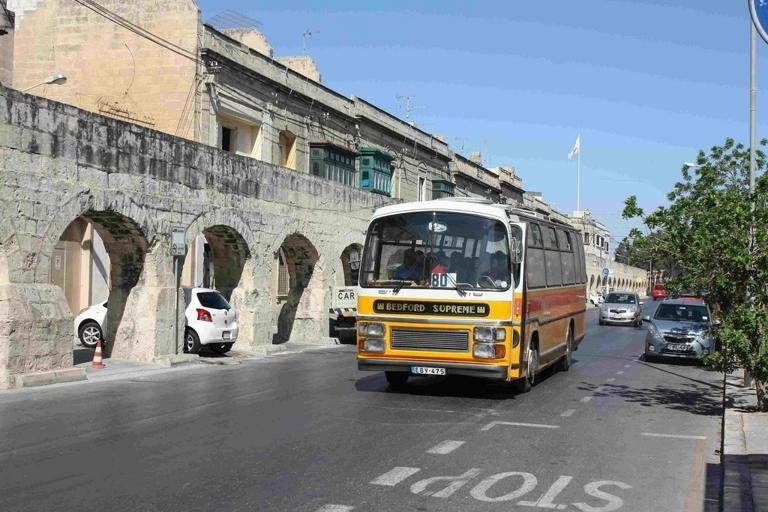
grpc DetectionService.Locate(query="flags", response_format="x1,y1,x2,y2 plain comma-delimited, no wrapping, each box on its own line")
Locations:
567,139,579,157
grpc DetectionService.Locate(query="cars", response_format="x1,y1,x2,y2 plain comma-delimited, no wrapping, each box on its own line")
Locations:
641,293,723,364
599,291,643,326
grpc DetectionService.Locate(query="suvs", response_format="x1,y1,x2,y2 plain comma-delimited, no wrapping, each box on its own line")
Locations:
75,286,237,355
653,283,668,298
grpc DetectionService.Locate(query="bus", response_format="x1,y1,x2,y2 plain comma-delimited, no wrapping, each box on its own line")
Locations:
353,196,588,392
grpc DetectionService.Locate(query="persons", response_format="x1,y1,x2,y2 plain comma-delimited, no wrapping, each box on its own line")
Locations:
394,249,509,285
